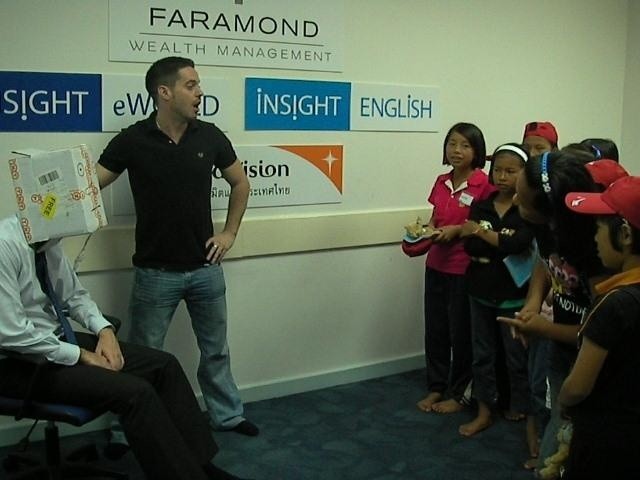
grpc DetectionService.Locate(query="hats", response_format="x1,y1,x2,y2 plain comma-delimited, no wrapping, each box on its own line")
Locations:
563,174,640,230
583,158,628,190
524,121,557,144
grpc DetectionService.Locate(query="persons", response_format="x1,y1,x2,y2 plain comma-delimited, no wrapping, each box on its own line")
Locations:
0,212,242,479
406,119,640,480
82,55,264,438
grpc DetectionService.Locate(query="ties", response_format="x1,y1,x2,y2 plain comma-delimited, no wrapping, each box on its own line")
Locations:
34,250,78,347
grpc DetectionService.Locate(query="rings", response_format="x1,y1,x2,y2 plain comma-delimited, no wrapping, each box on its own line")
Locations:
213,244,219,249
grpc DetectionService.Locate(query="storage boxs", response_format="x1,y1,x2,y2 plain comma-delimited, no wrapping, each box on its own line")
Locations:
9,144,108,253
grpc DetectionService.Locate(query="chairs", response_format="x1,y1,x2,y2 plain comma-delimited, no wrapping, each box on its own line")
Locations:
0,314,121,480
